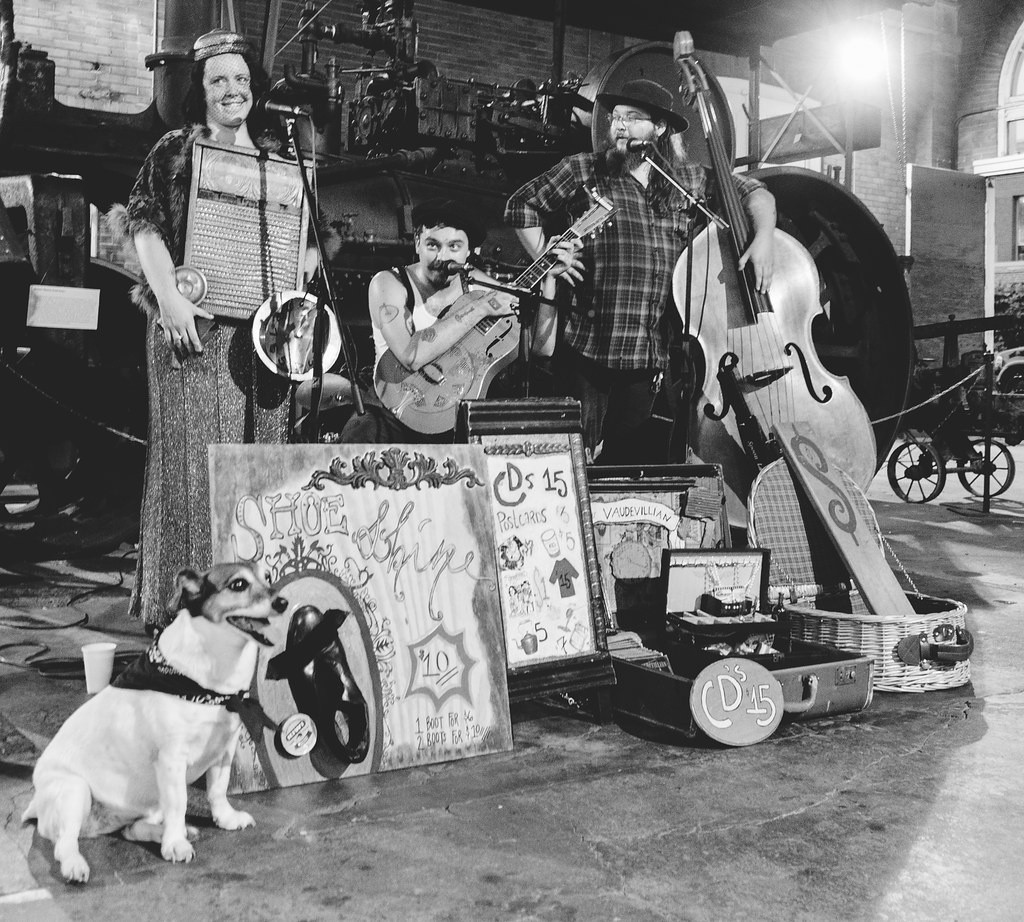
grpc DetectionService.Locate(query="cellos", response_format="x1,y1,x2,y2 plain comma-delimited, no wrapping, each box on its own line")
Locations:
670,26,879,530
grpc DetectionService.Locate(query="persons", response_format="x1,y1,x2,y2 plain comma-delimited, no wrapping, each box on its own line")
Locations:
109,27,341,637
503,79,776,466
341,199,587,442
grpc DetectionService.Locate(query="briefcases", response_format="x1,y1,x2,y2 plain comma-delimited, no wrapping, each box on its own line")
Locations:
585,461,875,740
745,456,973,693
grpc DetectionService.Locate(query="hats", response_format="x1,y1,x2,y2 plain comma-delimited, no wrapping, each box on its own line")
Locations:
595,79,689,136
193,28,250,60
411,200,486,247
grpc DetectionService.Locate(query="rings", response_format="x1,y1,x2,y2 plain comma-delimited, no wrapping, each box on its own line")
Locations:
172,335,183,341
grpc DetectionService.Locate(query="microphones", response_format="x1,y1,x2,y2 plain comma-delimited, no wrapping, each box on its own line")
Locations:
255,94,310,118
443,259,475,275
626,137,653,153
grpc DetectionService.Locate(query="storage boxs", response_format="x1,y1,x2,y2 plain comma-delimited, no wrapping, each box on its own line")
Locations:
656,545,796,670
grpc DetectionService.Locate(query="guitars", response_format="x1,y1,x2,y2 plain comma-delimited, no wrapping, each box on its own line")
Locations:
373,192,619,435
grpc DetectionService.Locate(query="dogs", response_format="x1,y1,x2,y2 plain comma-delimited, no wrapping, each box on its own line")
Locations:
18,563,287,884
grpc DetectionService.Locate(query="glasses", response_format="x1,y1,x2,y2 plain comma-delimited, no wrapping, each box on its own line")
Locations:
605,112,656,125
